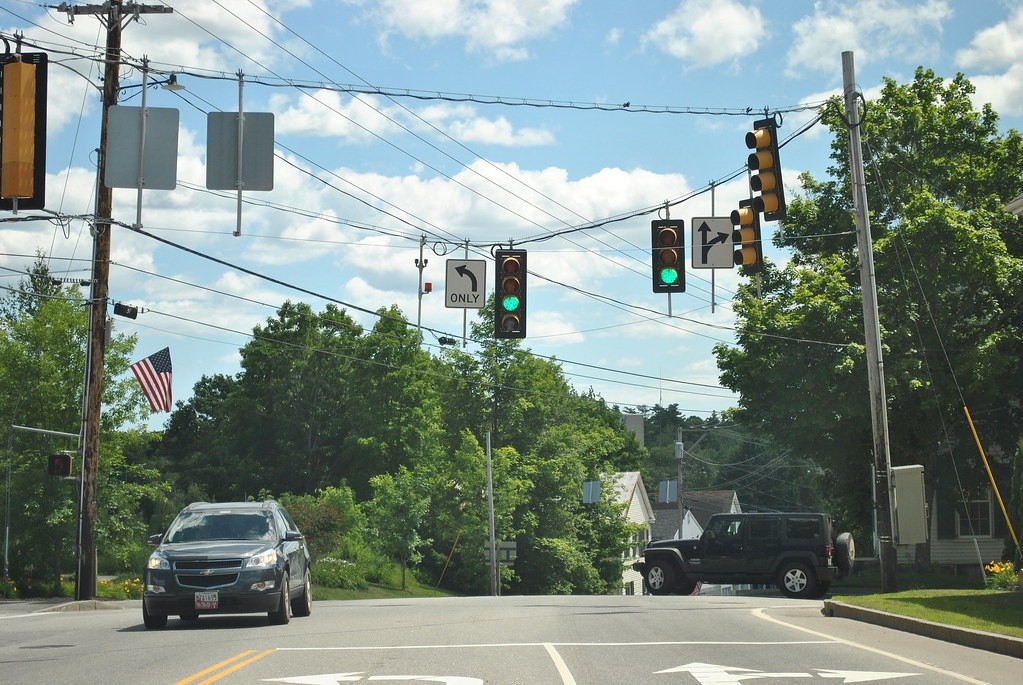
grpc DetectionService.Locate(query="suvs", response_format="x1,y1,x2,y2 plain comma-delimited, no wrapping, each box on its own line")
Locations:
632,513,856,600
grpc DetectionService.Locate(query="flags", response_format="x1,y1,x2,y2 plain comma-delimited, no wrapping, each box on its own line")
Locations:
130,346,173,413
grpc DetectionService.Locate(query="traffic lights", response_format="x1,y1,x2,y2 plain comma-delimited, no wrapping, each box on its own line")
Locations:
744,119,786,222
47,455,73,479
730,199,763,271
495,248,526,340
651,219,687,293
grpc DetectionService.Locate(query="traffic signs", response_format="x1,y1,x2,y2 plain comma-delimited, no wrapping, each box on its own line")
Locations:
446,259,487,307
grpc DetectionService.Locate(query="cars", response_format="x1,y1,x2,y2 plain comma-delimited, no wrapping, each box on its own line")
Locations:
142,499,315,630
